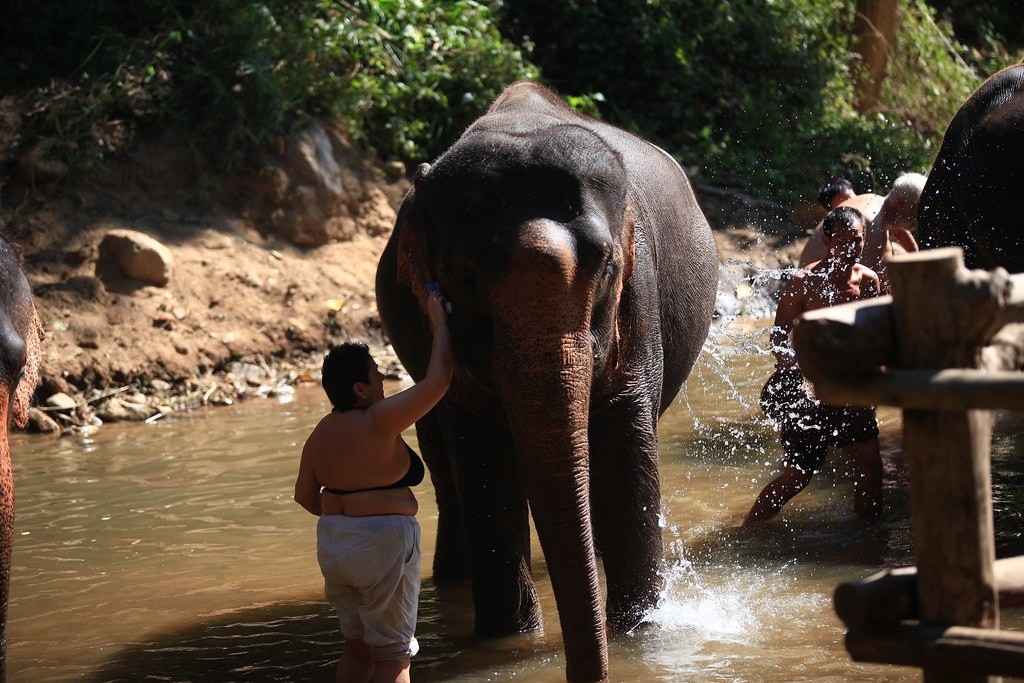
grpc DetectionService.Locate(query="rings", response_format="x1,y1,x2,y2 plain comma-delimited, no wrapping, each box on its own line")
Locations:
432,297,437,302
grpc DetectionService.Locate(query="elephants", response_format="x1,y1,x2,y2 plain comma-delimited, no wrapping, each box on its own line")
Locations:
374,81,720,682
916,53,1023,270
1,233,35,681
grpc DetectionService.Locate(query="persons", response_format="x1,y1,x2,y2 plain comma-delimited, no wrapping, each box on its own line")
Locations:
743,174,927,527
293,291,451,683
